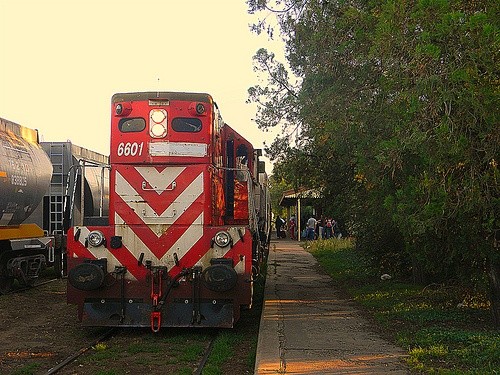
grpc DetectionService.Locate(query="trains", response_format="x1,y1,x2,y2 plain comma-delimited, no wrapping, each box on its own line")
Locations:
0,117,110,295
60,91,272,333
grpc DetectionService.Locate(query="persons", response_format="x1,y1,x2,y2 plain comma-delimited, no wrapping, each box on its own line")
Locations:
275,215,283,239
302,215,341,241
305,215,318,241
287,217,296,240
